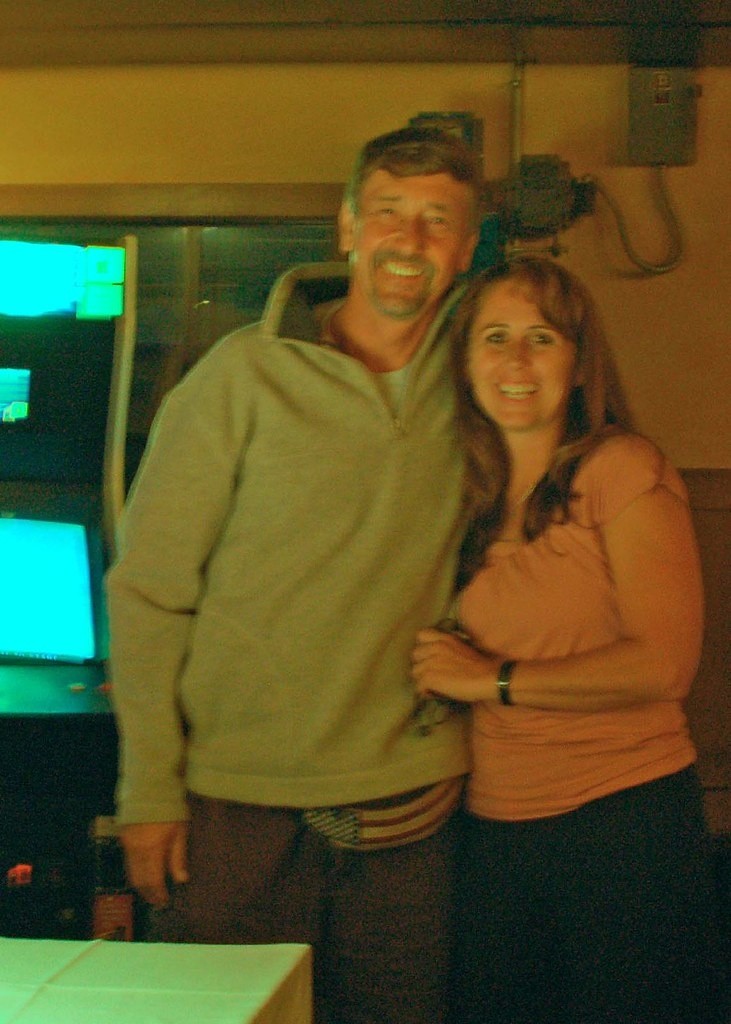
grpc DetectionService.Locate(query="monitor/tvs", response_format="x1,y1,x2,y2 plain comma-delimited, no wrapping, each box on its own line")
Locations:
0,517,96,662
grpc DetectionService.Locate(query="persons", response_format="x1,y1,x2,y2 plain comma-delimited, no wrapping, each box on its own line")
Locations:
411,254,720,1024
104,121,475,1024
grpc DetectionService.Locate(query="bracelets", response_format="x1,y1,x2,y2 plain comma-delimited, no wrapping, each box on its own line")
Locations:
495,659,516,705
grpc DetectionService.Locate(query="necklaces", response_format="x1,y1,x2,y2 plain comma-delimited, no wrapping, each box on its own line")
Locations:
517,477,539,508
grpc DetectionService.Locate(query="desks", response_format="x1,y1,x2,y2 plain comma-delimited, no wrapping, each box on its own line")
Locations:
0,937,315,1024
